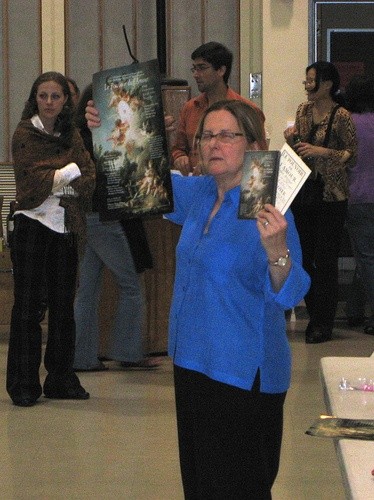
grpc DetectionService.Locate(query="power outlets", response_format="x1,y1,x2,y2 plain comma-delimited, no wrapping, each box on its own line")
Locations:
249,72,262,98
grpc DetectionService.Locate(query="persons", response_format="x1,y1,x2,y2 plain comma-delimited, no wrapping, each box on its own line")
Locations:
75,83,164,372
6,71,96,408
169,41,266,177
85,98,311,500
345,75,374,334
283,60,359,344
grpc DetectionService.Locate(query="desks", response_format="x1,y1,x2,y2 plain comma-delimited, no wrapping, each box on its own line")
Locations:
318,357,373,500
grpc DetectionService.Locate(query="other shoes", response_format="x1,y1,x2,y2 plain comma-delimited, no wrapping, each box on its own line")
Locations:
305,331,331,344
365,324,374,335
94,365,111,370
14,399,36,406
123,362,158,368
47,391,89,399
305,324,314,336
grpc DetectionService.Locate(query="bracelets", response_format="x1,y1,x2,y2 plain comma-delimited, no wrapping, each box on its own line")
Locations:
268,249,291,267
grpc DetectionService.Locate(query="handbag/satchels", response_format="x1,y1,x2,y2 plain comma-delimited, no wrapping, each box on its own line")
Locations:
291,179,324,225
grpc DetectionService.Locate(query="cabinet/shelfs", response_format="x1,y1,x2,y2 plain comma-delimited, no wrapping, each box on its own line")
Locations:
79,81,192,357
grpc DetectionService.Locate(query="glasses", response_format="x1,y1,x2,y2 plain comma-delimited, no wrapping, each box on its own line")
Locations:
197,130,248,142
190,66,209,73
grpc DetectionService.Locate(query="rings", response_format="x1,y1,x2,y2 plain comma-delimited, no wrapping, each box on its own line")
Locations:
263,221,269,227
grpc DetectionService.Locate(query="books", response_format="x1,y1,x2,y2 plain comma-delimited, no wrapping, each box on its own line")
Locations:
237,150,281,220
